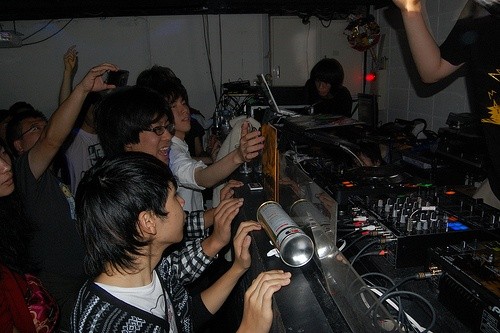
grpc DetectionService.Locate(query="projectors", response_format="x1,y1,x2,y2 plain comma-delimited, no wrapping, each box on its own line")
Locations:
0,30,21,48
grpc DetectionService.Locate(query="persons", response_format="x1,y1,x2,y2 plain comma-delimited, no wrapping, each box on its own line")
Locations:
0,101,36,144
59,149,291,333
148,77,265,211
304,58,352,115
394,0,500,209
58,46,104,198
0,142,60,333
137,65,220,164
8,62,118,333
91,90,245,284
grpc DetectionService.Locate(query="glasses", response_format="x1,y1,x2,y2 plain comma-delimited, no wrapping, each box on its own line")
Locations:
144,122,177,135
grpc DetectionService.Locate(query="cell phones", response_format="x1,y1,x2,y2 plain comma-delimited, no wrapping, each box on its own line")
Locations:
106,70,129,86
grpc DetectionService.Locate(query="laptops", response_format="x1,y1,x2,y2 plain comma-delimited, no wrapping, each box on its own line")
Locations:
256,74,298,116
